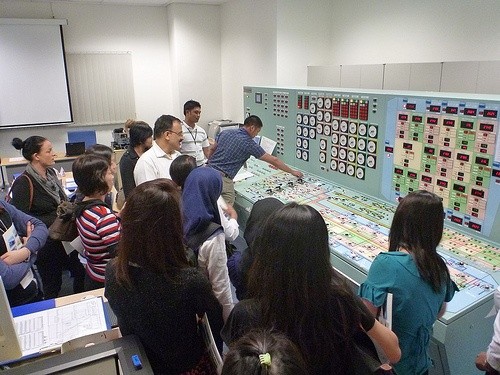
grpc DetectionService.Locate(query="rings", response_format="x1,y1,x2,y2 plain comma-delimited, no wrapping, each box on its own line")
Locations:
298,174,299,176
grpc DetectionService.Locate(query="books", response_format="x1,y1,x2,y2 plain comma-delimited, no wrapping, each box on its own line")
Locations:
3,221,34,289
70,236,86,258
376,292,393,331
199,312,224,375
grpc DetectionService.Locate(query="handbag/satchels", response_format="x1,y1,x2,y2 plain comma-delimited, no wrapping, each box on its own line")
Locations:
47,201,79,242
4,174,34,211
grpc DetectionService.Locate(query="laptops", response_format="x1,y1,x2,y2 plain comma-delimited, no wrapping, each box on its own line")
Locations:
66,142,85,157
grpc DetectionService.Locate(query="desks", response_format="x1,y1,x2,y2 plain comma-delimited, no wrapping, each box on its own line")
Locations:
0,287,123,369
0,148,127,190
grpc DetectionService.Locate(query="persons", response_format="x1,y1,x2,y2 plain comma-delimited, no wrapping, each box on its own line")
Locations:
119,120,153,203
227,198,285,301
475,286,500,375
180,167,234,325
207,115,303,206
103,178,224,375
357,190,460,375
222,329,307,375
0,200,48,308
170,155,239,259
73,153,126,291
133,115,183,187
220,202,402,375
180,100,210,168
11,136,86,300
75,144,118,213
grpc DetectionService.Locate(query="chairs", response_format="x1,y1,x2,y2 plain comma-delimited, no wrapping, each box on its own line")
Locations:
68,130,95,148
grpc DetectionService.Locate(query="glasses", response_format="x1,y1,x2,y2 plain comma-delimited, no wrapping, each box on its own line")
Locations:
165,129,182,137
